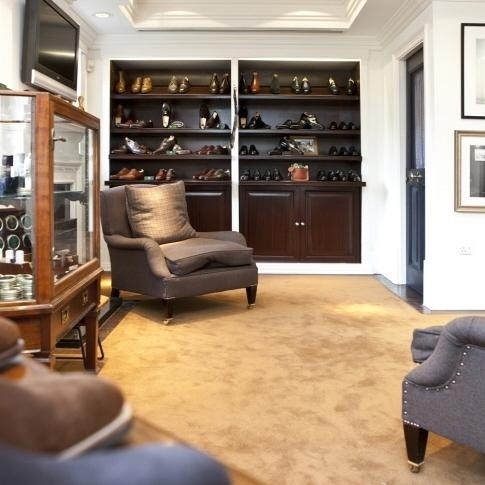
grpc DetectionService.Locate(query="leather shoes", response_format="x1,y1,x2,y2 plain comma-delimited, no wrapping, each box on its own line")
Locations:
110,72,358,180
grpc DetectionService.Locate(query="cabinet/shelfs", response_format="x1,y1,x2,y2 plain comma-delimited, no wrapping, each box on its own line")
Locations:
111,60,231,232
0,89,99,369
238,57,366,265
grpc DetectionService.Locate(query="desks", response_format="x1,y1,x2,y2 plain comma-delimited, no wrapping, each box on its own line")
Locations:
1,346,266,485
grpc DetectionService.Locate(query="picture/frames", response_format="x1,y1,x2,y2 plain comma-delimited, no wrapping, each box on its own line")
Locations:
460,23,485,119
454,130,485,212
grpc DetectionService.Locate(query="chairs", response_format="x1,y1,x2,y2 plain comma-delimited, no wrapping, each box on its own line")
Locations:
99,184,258,317
400,316,485,473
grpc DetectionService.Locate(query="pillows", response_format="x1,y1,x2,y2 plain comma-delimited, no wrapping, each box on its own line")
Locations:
124,180,198,243
